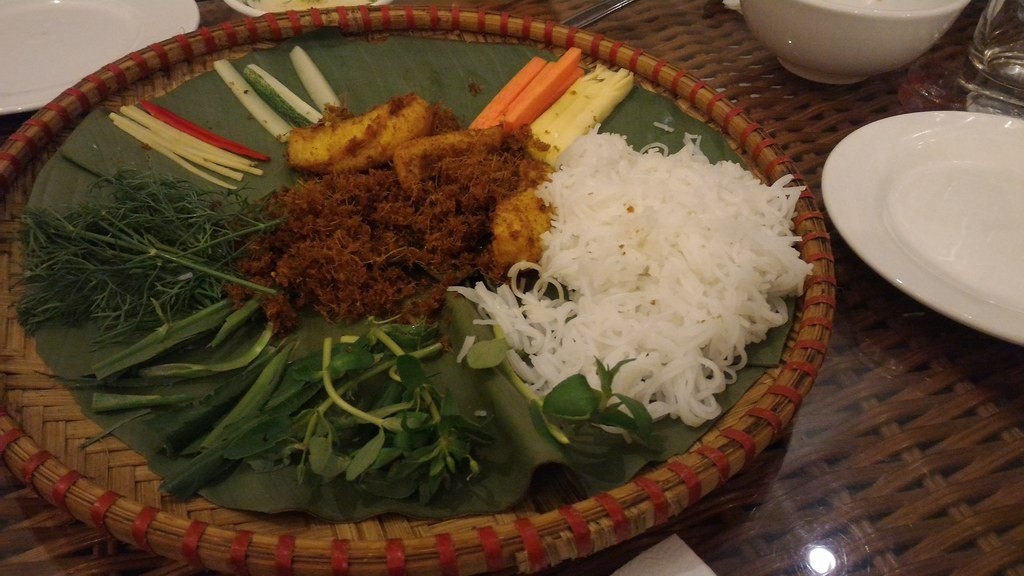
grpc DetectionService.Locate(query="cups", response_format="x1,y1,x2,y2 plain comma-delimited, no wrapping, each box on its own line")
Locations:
959,1,1024,122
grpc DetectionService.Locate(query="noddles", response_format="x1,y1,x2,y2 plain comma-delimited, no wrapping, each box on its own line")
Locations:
431,118,815,454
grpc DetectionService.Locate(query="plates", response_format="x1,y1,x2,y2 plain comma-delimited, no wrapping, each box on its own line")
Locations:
823,111,1024,346
0,0,202,116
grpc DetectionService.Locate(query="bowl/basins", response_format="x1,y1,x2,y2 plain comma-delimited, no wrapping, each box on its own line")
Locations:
741,0,971,85
